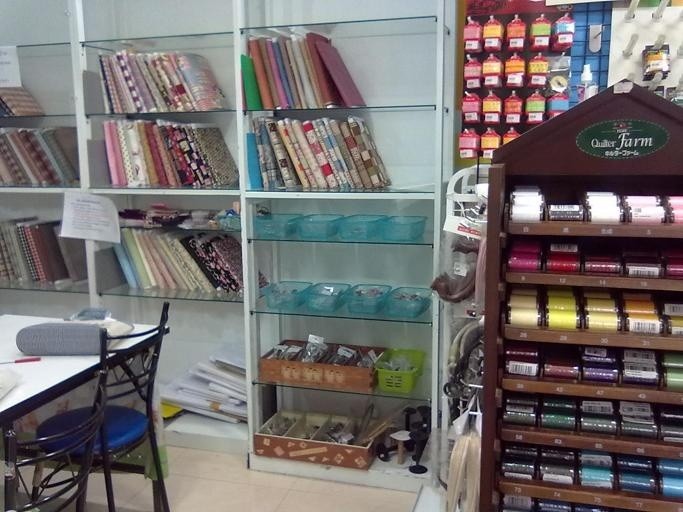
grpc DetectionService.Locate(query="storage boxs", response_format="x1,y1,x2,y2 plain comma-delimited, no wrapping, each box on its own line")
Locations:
256,215,427,241
257,339,383,394
265,281,431,318
254,410,382,471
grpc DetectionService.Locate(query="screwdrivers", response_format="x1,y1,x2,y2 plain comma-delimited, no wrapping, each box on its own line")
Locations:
0,357,40,364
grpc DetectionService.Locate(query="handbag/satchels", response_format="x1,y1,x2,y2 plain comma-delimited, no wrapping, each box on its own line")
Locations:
16,307,134,356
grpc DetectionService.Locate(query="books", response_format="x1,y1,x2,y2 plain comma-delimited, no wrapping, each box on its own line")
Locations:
157,341,248,425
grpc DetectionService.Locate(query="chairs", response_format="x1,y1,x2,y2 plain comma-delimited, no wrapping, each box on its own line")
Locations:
32,303,168,512
5,370,109,512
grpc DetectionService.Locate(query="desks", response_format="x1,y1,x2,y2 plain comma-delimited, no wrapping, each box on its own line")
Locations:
1,314,170,511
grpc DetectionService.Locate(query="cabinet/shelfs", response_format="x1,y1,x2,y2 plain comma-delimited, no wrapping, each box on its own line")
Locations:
236,2,443,490
77,1,249,463
1,0,94,318
480,77,683,512
